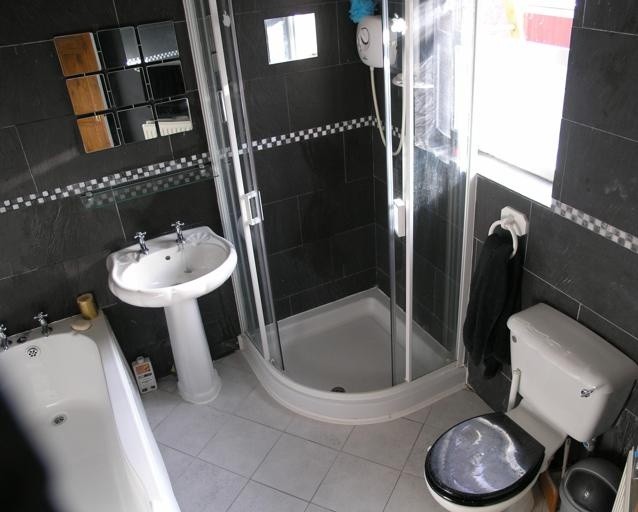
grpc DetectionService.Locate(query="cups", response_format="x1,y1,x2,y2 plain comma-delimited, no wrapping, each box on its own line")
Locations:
78,293,98,322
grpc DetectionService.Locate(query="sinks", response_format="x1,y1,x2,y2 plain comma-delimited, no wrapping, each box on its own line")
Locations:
106,226,237,308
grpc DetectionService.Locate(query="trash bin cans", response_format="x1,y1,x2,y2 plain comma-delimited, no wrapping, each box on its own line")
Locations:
557,458,623,512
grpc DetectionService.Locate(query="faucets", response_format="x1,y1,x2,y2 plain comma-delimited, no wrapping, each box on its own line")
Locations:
134,231,149,254
170,221,186,242
0,324,12,351
34,312,53,337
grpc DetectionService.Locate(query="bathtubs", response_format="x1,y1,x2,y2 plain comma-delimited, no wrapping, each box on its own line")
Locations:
0,309,181,511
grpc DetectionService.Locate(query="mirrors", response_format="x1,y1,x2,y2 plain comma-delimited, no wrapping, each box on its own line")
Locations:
263,11,318,66
51,20,195,155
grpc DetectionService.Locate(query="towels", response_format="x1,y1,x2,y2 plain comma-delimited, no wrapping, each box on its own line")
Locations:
463,231,532,380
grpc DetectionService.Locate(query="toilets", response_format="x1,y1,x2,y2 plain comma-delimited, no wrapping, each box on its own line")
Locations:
424,303,638,511
